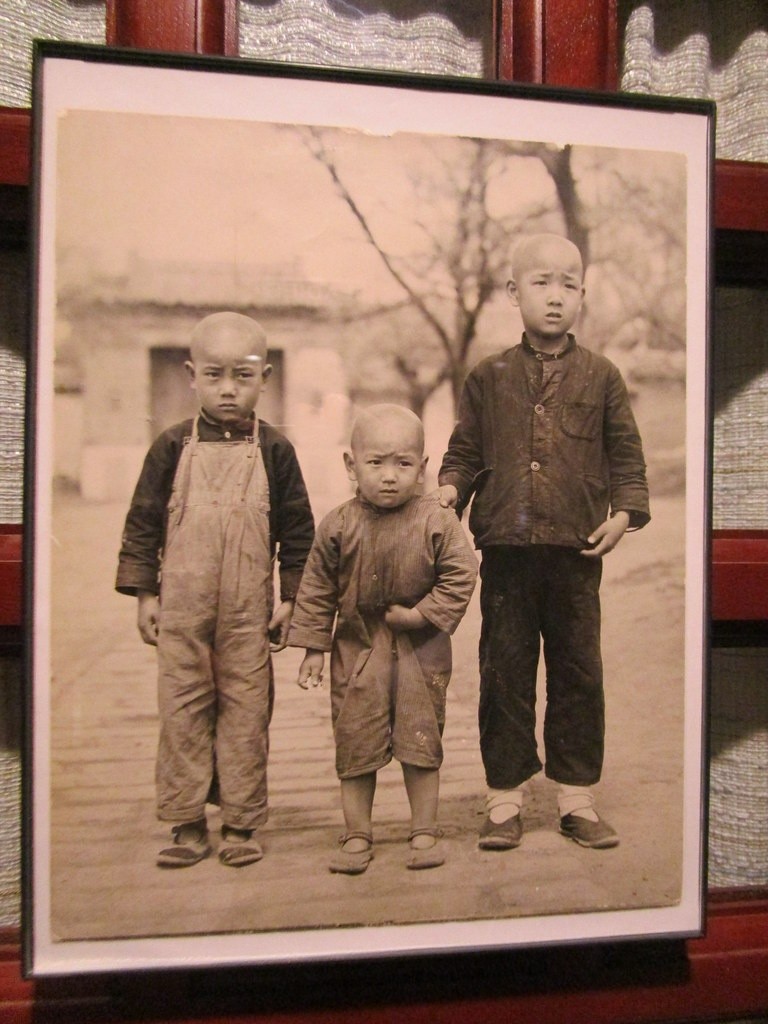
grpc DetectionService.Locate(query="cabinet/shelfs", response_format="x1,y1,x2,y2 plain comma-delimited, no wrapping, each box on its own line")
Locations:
0,0,767,1024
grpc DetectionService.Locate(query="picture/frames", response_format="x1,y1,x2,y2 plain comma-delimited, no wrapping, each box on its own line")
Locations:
23,38,718,981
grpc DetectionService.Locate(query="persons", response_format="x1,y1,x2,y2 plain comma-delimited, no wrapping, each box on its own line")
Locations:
116,311,315,869
438,235,641,849
286,401,477,870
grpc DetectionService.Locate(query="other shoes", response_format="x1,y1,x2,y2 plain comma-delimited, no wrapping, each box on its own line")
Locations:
405,825,447,870
217,828,264,864
478,810,524,849
158,829,212,865
329,826,375,876
559,806,621,849
